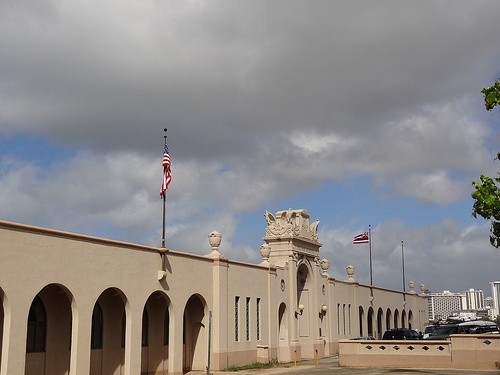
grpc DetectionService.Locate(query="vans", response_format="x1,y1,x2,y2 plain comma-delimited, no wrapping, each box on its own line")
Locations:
424,316,499,340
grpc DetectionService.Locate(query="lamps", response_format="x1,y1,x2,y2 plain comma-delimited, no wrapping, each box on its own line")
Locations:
295,304,304,318
319,305,327,319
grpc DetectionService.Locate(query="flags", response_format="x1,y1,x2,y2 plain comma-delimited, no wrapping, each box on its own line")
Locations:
159,144,172,198
353,231,370,244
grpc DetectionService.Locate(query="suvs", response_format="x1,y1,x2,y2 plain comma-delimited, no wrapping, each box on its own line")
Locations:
382,327,422,340
